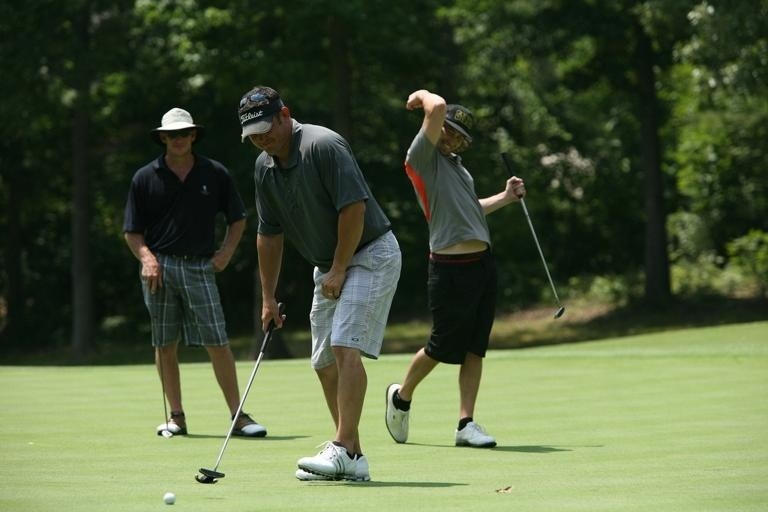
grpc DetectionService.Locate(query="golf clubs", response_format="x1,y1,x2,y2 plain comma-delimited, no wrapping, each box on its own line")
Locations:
195,300,285,483
501,150,564,318
161,285,174,438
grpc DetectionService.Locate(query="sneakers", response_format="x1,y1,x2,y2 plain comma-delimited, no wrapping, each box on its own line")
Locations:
456,422,496,447
232,414,266,436
385,384,409,443
158,411,187,435
295,442,369,481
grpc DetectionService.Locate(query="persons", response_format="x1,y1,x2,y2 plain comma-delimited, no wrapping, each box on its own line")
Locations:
122,106,269,436
385,89,527,447
237,85,402,482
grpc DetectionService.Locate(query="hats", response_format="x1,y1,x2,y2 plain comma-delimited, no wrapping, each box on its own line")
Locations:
444,104,474,140
157,108,196,130
238,98,284,143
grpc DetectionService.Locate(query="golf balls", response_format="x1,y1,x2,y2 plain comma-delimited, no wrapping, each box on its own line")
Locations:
163,492,176,505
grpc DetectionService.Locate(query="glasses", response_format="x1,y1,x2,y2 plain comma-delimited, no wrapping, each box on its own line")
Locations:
239,94,265,108
168,130,190,139
